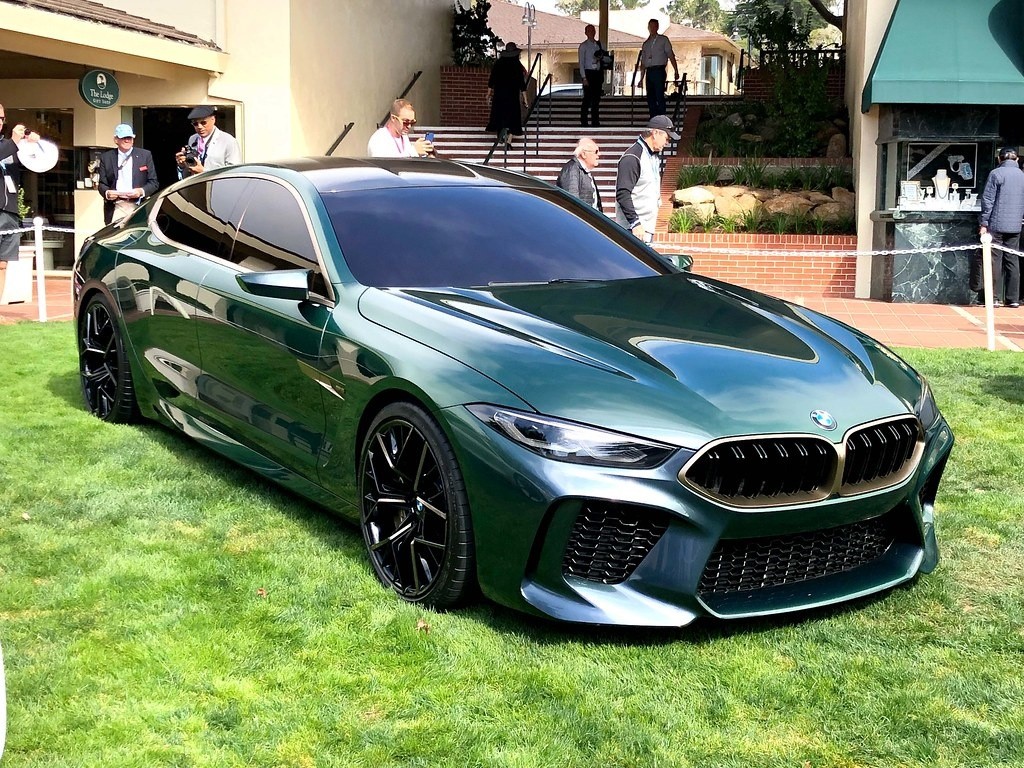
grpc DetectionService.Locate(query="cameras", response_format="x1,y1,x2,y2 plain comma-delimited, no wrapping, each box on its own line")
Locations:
24,128,31,137
180,145,200,165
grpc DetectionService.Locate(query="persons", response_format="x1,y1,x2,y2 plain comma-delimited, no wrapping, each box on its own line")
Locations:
367,99,437,157
98,124,159,226
485,42,529,149
557,138,603,214
615,115,681,246
0,104,41,303
176,107,241,180
979,146,1024,308
637,19,679,120
578,25,608,126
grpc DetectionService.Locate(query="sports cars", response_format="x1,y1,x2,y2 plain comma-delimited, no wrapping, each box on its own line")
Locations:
72,155,955,629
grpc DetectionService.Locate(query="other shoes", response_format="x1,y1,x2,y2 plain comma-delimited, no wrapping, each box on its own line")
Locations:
993,300,1000,308
1005,303,1020,308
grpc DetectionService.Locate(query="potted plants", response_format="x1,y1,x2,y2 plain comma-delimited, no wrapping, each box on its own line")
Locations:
0,184,36,304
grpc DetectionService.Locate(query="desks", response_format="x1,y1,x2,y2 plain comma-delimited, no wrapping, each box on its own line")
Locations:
22,240,66,269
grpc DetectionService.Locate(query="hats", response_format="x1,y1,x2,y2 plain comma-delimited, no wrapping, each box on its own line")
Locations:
188,106,214,119
114,124,136,138
999,148,1015,157
647,115,680,140
501,42,523,58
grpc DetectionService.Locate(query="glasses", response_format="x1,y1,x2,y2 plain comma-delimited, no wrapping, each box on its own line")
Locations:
664,127,676,132
192,121,207,127
0,117,5,120
584,150,599,155
395,116,417,125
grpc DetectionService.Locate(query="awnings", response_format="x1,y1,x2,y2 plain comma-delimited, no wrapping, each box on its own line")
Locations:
861,0,1024,113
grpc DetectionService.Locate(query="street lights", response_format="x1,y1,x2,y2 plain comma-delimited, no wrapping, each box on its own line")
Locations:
522,1,537,74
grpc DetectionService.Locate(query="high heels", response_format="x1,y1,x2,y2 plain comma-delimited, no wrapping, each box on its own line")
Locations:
507,144,514,151
499,137,505,144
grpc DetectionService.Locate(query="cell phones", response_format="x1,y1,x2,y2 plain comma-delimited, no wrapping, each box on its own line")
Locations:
425,133,434,143
